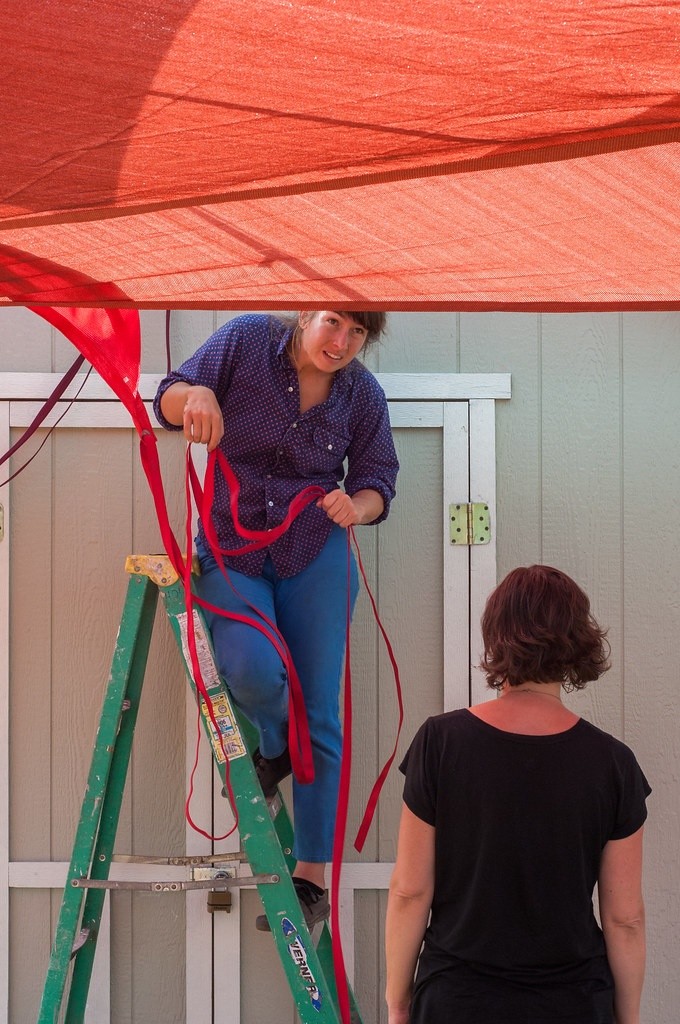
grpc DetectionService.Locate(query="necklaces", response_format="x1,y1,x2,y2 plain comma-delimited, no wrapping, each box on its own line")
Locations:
505,688,566,703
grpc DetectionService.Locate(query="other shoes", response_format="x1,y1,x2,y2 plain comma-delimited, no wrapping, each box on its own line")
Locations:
256,876,331,931
221,744,294,799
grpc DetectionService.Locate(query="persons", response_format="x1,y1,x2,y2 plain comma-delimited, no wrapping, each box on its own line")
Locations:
145,304,402,937
377,558,651,1023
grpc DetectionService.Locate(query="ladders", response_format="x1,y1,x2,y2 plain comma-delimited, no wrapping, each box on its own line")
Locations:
38,554,362,1022
